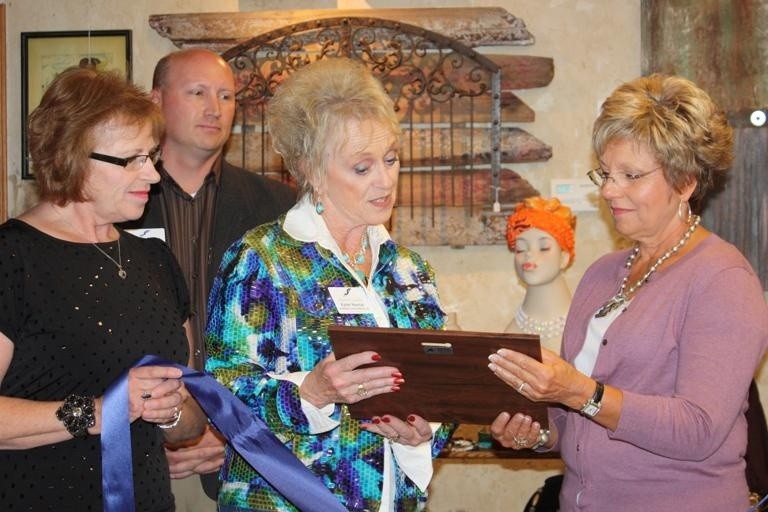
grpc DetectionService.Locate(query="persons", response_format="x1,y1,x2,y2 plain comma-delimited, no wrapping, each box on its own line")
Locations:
0,67,209,512
202,58,459,512
490,73,764,512
116,48,301,512
502,197,577,357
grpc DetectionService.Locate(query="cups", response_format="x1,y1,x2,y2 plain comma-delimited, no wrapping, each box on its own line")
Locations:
85,147,165,172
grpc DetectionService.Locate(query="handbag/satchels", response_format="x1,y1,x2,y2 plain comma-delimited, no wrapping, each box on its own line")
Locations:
156,409,182,429
533,428,551,450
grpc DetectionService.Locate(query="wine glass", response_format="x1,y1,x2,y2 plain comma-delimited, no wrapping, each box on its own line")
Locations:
141,393,152,400
174,406,178,417
392,433,402,440
518,383,525,393
515,437,529,446
356,384,367,398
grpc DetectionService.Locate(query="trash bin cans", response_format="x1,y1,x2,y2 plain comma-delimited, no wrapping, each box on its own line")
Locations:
581,382,606,419
57,392,96,440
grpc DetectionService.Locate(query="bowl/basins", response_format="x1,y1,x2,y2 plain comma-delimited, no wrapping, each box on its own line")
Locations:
46,200,127,279
595,214,702,320
342,235,369,283
516,304,568,342
